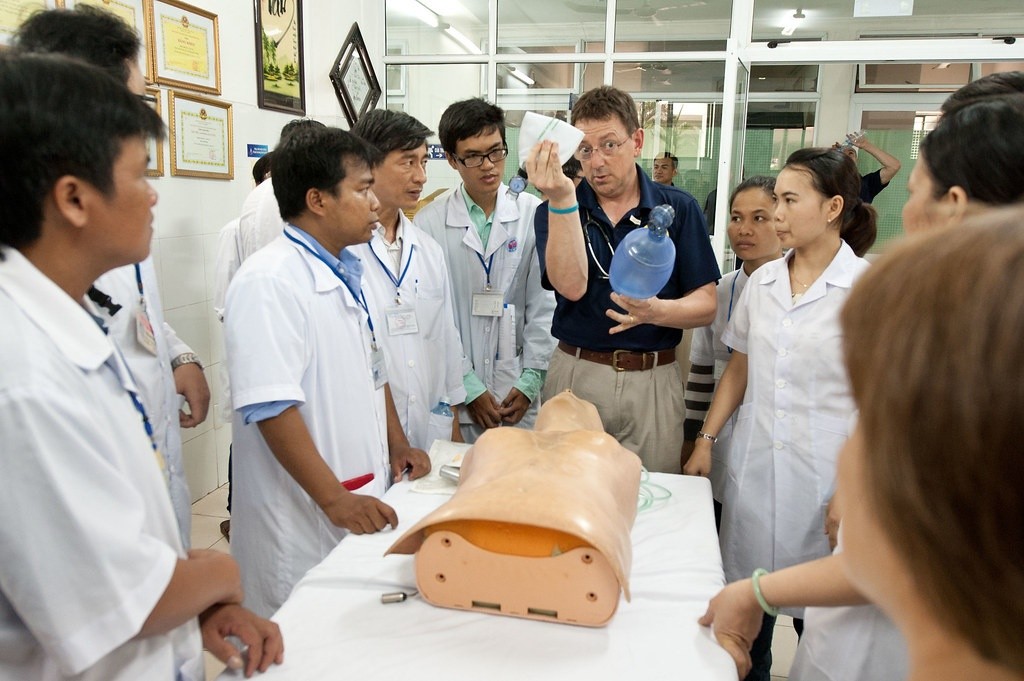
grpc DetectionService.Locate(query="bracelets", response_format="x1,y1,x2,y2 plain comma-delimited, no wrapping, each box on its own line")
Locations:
171,353,204,372
697,431,718,445
548,202,578,215
752,568,778,615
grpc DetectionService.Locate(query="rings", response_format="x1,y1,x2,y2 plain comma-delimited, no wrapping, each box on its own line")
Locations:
629,315,634,324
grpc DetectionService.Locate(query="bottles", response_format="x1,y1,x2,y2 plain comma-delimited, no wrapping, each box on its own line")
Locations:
836,129,870,152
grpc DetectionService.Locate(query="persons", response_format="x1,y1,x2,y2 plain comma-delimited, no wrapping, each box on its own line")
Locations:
0,5,284,680
384,389,643,628
213,69,1024,681
653,151,679,186
830,131,901,205
702,163,745,237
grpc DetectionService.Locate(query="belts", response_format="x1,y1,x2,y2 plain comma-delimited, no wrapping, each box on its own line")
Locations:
558,339,675,370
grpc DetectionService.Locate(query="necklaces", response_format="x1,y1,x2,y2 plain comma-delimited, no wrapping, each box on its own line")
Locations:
608,215,617,224
793,272,812,288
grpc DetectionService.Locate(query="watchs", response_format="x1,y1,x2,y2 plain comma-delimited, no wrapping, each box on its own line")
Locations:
505,168,529,201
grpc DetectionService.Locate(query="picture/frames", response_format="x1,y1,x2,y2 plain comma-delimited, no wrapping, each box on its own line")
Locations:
169,91,234,180
330,22,383,129
150,0,221,94
139,87,164,177
253,0,306,115
57,0,154,84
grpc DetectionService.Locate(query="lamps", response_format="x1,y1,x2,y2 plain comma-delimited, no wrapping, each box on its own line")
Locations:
394,0,439,28
781,10,805,36
444,24,482,55
507,67,536,85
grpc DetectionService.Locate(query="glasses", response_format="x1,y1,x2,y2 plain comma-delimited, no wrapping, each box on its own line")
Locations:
450,141,508,168
134,92,158,110
573,130,635,160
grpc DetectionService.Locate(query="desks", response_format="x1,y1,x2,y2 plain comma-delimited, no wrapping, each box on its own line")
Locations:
208,441,738,680
